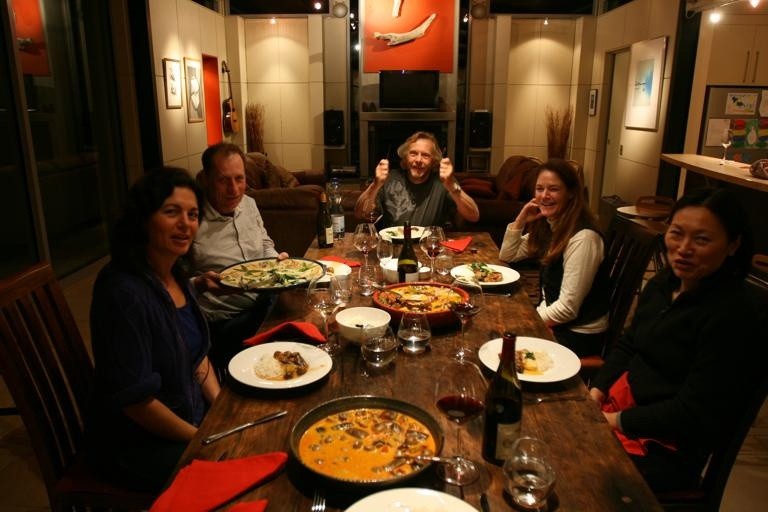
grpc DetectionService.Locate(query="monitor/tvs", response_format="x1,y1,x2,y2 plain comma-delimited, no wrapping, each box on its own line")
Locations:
379,69,440,111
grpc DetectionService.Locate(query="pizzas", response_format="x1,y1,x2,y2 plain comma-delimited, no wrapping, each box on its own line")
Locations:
220,258,323,288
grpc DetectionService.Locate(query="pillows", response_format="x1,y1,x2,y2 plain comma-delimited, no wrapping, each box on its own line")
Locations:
272,160,297,191
459,176,494,197
260,156,283,189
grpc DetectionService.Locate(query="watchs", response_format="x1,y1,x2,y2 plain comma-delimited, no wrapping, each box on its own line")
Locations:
450,183,462,195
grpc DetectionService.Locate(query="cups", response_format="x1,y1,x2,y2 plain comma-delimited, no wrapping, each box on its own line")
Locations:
360,199,384,224
502,437,557,509
307,222,485,371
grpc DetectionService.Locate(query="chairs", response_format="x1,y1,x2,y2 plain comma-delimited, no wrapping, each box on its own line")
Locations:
577,213,664,387
0,260,152,512
239,151,326,254
665,267,766,512
453,152,546,230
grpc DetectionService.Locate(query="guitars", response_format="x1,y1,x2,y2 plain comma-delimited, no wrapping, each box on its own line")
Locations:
222,60,240,135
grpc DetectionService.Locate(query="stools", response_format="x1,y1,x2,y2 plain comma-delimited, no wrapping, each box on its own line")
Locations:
615,202,671,302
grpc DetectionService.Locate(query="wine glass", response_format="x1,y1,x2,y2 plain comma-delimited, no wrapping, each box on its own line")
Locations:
434,361,488,485
720,129,734,165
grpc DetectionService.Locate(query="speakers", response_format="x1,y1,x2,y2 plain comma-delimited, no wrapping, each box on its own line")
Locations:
470,112,491,147
324,110,344,145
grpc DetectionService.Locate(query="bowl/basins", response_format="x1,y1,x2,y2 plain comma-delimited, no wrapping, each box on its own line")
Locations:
289,395,446,487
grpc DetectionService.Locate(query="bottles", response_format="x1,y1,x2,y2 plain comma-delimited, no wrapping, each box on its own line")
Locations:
316,191,334,247
482,331,525,467
327,175,346,239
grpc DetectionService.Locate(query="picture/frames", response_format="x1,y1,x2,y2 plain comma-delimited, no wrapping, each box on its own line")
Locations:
161,56,183,109
587,87,597,116
183,57,207,124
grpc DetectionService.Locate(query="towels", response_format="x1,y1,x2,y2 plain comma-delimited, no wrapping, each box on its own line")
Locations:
240,317,326,346
439,233,474,253
598,365,678,459
316,253,361,268
144,434,290,511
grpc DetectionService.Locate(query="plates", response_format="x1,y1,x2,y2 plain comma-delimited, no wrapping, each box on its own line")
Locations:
215,256,325,293
228,340,334,389
341,487,481,512
450,264,520,286
616,206,643,216
478,336,582,385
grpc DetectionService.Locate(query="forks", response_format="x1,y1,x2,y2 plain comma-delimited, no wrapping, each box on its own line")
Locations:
523,396,585,404
310,490,327,512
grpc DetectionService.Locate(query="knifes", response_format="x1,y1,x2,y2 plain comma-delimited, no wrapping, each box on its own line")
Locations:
202,410,288,443
480,493,490,512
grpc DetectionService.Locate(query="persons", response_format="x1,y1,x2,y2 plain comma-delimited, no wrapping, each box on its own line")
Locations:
498,159,611,358
186,141,289,365
88,167,230,492
585,187,746,487
352,130,480,232
